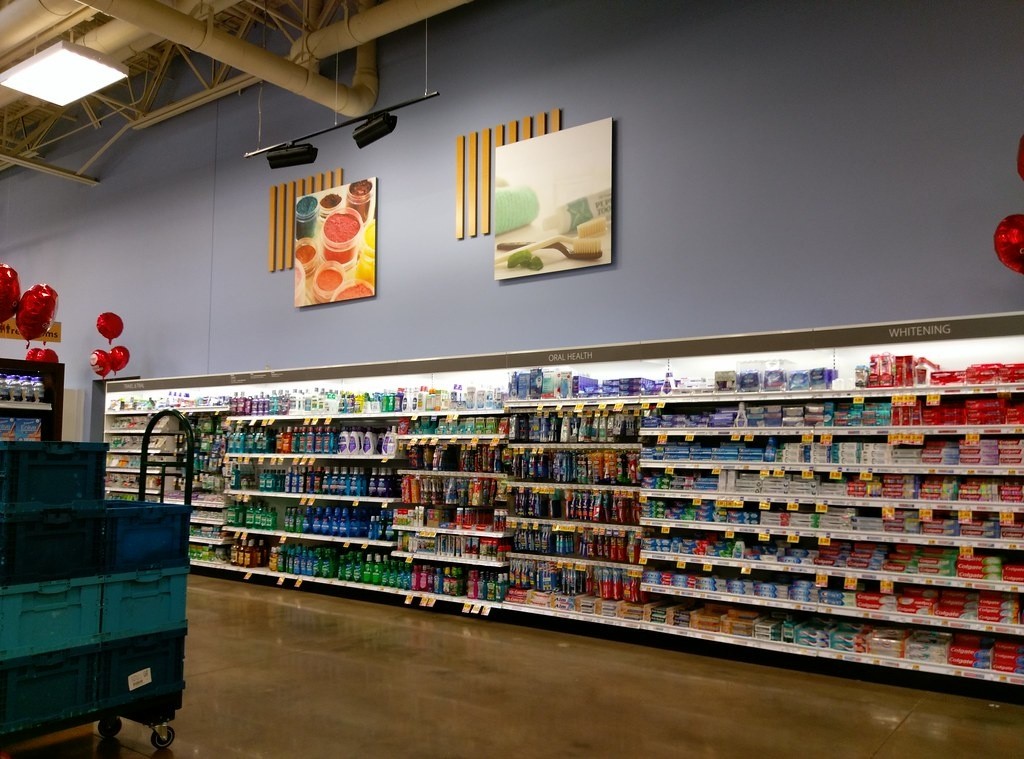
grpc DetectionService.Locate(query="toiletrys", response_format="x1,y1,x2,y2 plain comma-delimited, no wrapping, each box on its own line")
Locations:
92,351,1024,685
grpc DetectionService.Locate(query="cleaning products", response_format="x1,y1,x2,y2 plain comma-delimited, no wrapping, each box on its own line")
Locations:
0,365,51,448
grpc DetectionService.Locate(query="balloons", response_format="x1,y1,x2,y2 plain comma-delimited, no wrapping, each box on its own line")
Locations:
994,214,1024,274
0,262,21,324
90,350,111,380
15,283,59,349
111,346,130,375
97,312,124,344
26,349,59,363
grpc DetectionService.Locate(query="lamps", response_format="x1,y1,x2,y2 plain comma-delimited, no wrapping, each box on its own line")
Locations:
352,113,399,149
267,139,321,168
0,27,131,105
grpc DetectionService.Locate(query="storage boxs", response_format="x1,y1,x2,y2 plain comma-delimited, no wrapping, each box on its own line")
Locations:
2,433,188,746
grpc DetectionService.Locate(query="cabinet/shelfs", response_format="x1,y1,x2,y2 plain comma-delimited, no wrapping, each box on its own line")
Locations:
103,313,1024,692
1,357,66,448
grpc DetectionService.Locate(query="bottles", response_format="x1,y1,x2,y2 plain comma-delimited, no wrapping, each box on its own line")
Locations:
115,343,511,606
0,372,46,406
